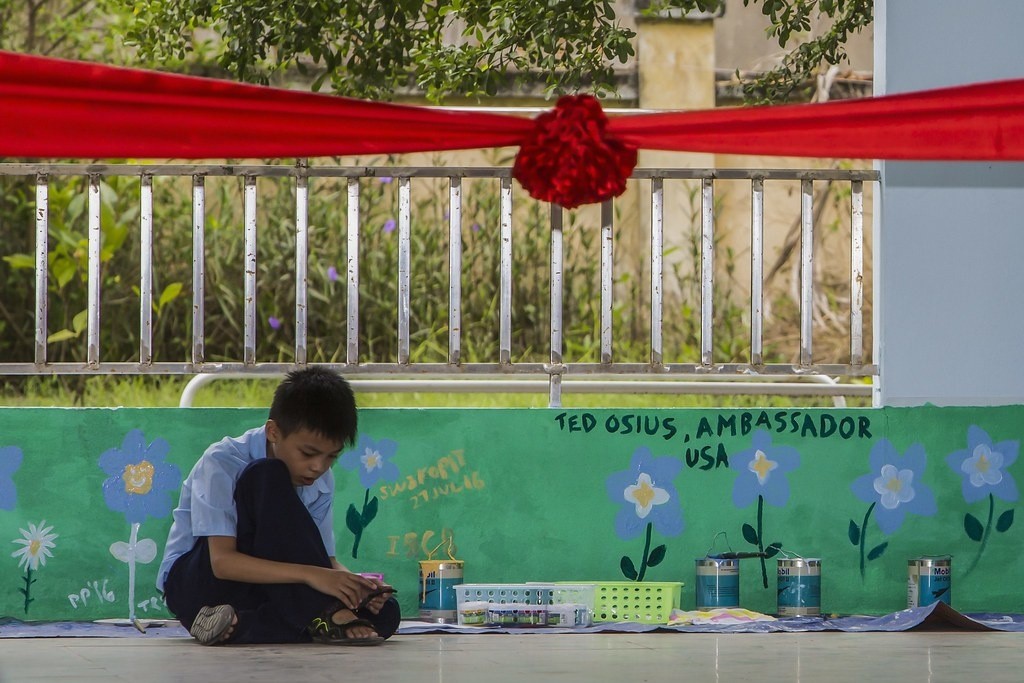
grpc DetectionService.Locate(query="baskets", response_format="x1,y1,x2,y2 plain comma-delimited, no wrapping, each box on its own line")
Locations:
452,581,683,631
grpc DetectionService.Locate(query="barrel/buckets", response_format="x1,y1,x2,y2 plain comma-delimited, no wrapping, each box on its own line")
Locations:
770,546,822,617
695,531,739,612
907,553,954,610
417,536,465,620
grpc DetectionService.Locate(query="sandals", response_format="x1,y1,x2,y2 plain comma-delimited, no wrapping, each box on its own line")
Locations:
306,604,384,645
190,604,239,646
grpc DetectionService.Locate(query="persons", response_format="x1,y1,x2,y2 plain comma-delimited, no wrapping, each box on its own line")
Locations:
156,365,401,647
